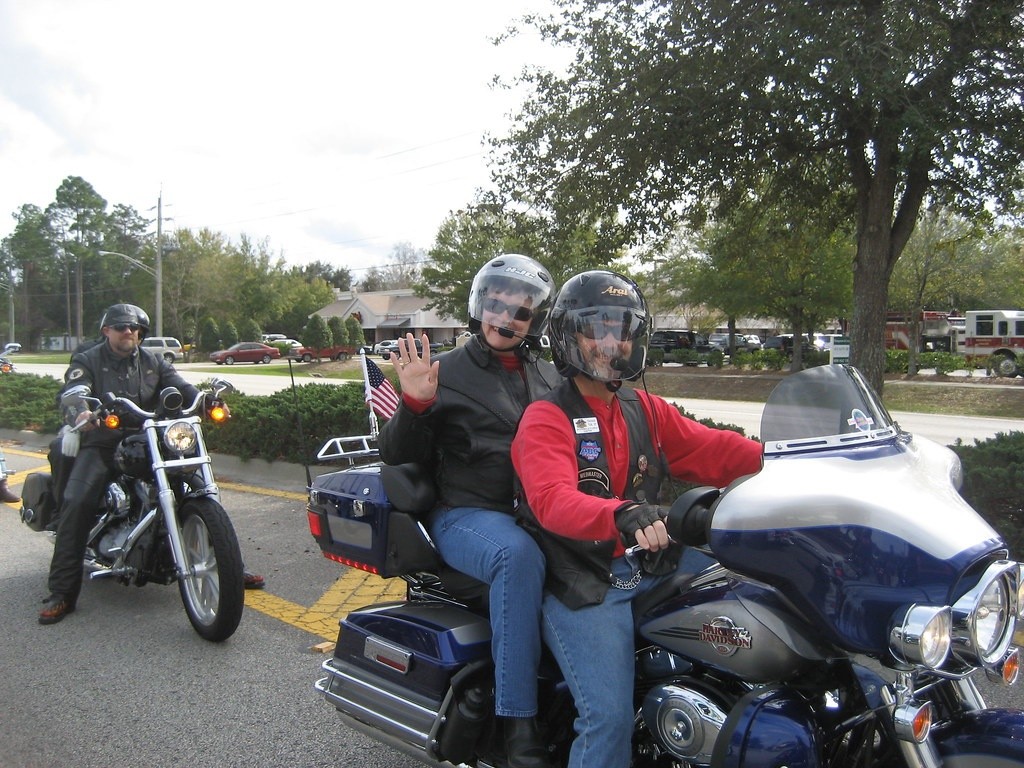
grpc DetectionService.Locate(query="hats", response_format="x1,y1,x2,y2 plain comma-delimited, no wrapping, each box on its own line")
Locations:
105,304,138,328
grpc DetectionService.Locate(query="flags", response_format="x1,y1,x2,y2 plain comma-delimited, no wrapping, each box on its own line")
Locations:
364,356,400,422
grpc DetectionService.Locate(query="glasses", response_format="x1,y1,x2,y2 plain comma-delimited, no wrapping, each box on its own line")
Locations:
138,334,142,339
580,322,635,341
109,323,139,331
484,296,532,321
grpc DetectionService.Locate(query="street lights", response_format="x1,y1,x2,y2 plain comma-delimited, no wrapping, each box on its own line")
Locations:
98,250,163,337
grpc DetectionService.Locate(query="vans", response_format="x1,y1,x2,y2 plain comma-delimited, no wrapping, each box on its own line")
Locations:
966,310,1024,378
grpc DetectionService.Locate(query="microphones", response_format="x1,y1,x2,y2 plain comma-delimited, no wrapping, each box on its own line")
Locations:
498,326,534,345
610,358,639,378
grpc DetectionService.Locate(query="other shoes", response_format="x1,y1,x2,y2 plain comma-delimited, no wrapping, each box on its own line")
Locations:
0,479,20,502
46,518,60,531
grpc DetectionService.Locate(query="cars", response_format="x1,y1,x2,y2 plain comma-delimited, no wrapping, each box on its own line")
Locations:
706,331,852,365
360,339,445,361
260,333,303,348
208,342,280,365
287,341,357,362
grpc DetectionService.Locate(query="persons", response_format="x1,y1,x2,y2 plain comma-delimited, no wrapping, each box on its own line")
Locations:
510,270,764,767
0,450,21,502
376,252,567,767
37,303,266,626
45,304,150,532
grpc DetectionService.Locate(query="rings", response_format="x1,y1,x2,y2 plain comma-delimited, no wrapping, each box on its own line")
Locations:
403,361,410,366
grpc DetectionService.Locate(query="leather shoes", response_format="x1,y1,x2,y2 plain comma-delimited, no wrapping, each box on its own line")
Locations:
504,718,552,768
244,571,265,589
39,594,74,623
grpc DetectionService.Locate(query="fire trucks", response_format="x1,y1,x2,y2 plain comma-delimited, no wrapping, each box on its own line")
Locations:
882,310,966,354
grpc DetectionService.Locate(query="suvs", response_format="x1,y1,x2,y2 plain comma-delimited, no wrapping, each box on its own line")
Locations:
139,337,184,363
649,329,726,367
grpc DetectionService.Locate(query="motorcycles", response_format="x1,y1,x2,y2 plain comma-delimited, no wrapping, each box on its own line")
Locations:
304,363,1024,768
51,379,246,643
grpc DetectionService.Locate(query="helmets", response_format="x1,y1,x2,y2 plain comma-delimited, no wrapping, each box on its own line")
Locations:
468,254,556,346
549,270,654,382
98,304,149,331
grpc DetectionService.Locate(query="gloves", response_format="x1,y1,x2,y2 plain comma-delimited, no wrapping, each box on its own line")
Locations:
61,425,80,457
